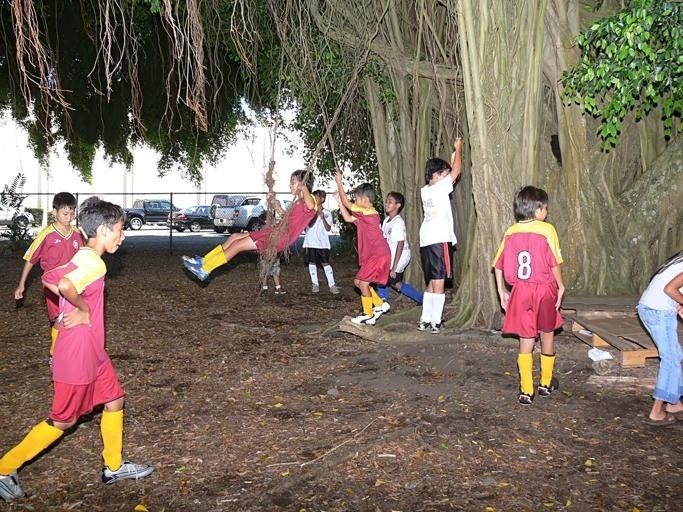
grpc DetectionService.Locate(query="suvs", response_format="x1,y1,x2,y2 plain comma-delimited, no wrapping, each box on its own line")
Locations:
209,194,253,234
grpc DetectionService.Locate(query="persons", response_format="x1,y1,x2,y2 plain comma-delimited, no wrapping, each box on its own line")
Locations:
413,137,463,333
300,190,340,295
0,198,155,504
12,191,88,372
489,184,566,408
375,191,424,306
331,168,391,326
635,248,682,426
180,171,318,284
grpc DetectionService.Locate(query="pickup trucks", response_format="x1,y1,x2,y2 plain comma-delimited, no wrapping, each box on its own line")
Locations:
214,197,292,233
120,198,183,229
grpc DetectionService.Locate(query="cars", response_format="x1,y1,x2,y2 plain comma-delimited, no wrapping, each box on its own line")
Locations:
0,201,34,230
166,203,213,232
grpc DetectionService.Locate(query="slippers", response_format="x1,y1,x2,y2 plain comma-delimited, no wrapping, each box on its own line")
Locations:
645,408,683,426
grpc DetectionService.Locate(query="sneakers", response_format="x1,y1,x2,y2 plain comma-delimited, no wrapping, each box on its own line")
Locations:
519,377,558,405
0,472,27,502
417,322,440,334
351,300,390,325
312,284,319,293
102,460,155,485
182,255,209,282
274,288,286,294
329,285,340,294
